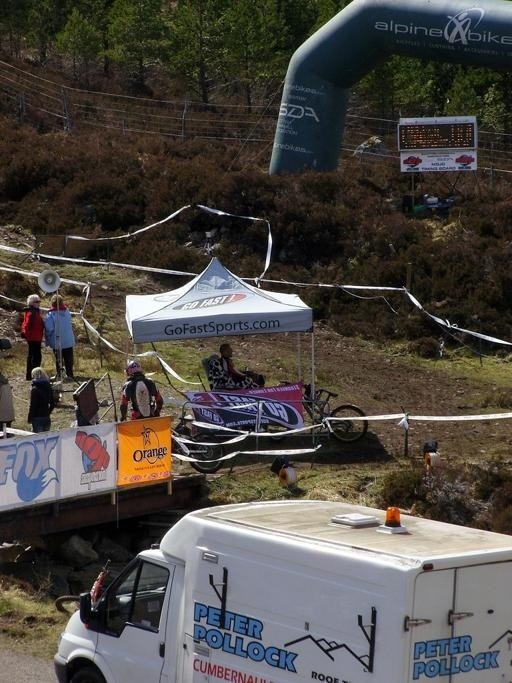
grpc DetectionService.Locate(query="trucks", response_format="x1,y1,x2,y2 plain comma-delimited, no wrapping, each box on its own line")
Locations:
53,499,511,683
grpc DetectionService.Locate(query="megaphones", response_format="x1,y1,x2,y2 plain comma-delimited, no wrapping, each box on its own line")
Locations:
37,269,61,293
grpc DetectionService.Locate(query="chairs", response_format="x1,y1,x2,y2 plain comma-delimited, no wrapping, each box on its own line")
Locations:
202,355,261,390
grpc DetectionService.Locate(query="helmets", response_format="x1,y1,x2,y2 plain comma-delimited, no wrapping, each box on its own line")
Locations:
126,361,144,376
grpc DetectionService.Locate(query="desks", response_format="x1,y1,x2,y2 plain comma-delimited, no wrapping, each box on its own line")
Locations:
423,192,454,220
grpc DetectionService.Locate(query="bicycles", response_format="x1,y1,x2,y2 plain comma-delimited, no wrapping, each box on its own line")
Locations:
267,383,368,441
169,417,227,472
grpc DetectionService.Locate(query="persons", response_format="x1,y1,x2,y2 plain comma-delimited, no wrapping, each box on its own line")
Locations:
209,343,265,388
20,295,44,381
43,295,76,382
28,367,55,433
0,372,15,439
119,360,163,422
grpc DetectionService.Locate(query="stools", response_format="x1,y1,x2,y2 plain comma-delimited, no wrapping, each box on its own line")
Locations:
402,194,416,212
